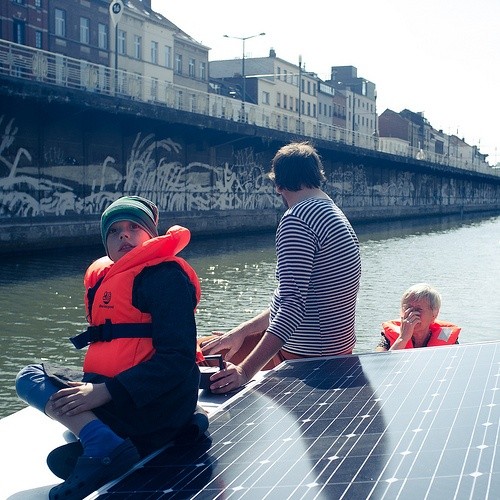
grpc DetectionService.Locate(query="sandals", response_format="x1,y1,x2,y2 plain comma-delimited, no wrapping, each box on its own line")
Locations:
46,441,83,481
48,438,141,500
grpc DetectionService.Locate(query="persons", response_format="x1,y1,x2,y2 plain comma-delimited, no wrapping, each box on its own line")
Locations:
14,195,210,500
375,281,460,354
190,141,361,396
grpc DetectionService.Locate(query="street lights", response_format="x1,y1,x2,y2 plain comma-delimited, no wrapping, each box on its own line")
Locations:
222,31,267,121
337,79,366,145
404,114,427,157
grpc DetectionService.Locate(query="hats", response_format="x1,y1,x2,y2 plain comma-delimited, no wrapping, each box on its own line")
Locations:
100,196,159,262
